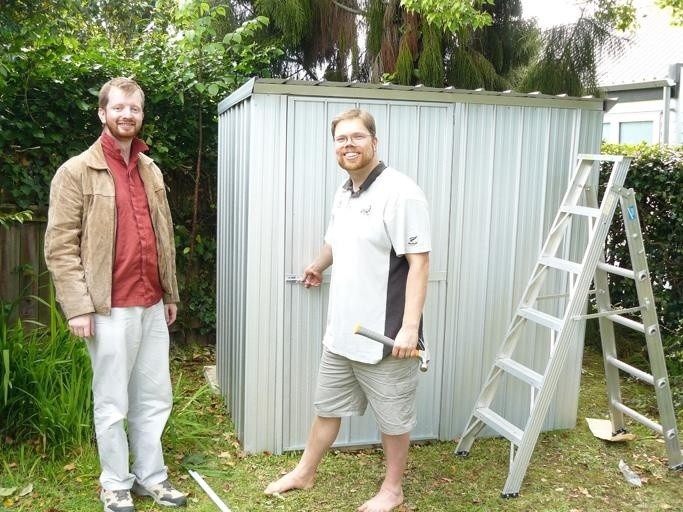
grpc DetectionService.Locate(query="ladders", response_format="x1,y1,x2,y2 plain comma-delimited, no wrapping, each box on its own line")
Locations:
453,154,683,499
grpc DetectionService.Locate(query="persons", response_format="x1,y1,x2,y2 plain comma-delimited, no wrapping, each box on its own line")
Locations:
262,108,433,512
41,76,191,511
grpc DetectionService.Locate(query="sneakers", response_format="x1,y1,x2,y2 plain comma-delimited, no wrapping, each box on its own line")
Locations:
135,480,187,507
99,487,135,512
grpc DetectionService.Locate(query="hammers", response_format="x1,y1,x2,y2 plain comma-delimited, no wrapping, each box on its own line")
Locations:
354,325,431,372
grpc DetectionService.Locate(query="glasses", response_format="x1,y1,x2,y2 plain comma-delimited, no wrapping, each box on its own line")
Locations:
335,134,374,144
